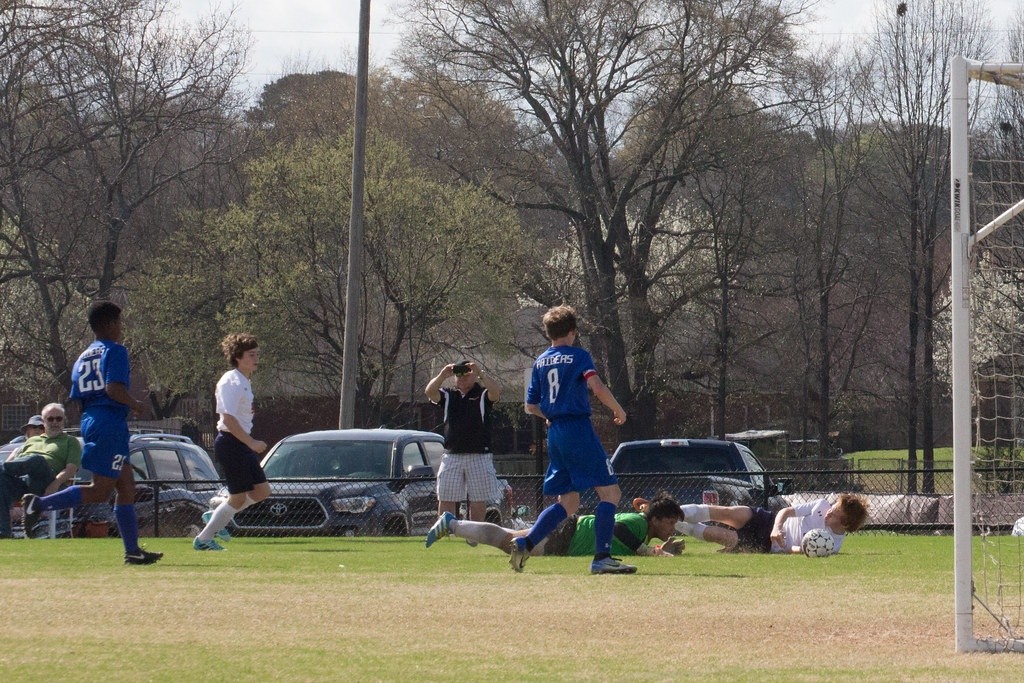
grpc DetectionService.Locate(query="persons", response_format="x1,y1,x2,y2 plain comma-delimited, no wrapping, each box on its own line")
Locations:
192,333,272,552
424,359,500,547
633,493,869,554
21,300,164,565
509,304,639,574
0,403,82,539
425,491,686,555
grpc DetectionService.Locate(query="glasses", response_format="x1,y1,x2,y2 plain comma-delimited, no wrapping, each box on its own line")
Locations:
27,425,44,429
44,416,64,422
456,372,470,376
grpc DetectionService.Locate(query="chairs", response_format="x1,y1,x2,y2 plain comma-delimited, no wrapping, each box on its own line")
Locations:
288,454,321,477
704,462,731,477
9,472,82,539
633,460,671,472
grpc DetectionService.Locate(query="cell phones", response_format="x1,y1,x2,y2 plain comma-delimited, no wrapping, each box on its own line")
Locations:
453,366,471,374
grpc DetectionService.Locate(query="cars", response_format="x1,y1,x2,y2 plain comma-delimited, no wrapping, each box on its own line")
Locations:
224,428,514,540
0,425,225,538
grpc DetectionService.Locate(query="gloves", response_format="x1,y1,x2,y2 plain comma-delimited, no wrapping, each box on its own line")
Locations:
659,536,685,555
638,541,672,556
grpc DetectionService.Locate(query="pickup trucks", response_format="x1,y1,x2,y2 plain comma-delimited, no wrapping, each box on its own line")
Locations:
571,438,795,520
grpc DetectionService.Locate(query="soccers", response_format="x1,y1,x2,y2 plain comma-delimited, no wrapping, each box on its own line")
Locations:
801,528,835,558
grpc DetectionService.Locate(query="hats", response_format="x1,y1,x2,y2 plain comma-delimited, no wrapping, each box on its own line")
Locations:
19,416,45,434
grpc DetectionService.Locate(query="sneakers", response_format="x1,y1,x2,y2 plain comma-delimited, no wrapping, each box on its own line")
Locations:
123,548,163,566
203,509,231,543
633,497,651,513
425,511,457,548
509,535,530,571
193,533,226,551
22,493,42,539
590,556,638,575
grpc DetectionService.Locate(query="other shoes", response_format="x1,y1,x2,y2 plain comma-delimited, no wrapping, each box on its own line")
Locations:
465,537,478,548
